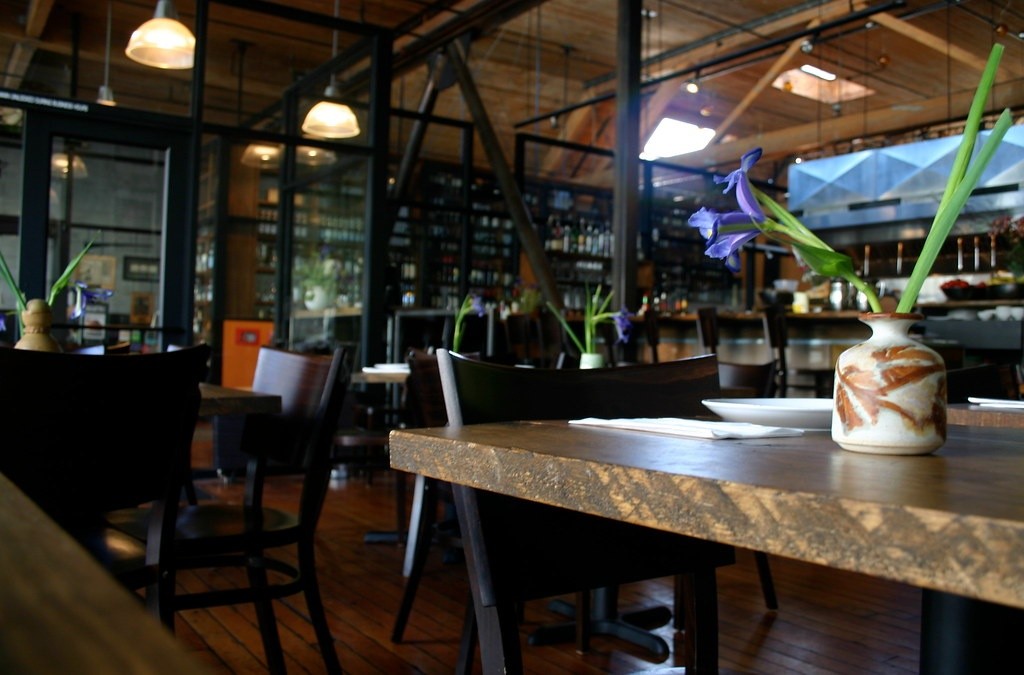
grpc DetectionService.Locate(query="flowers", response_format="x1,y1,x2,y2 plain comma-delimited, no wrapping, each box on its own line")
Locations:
688,44,1015,312
546,280,630,353
0,231,116,321
453,291,486,352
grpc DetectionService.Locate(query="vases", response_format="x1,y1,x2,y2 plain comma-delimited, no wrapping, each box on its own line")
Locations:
579,353,604,369
832,313,947,455
13,299,64,353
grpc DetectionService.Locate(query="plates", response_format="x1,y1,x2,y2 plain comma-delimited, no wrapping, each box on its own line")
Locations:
703,397,833,431
941,283,1024,299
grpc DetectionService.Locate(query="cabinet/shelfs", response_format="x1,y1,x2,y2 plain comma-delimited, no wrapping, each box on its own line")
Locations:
192,140,745,358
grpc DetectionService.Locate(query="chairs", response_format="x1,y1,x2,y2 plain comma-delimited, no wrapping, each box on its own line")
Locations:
0,343,210,639
107,345,354,675
436,347,737,675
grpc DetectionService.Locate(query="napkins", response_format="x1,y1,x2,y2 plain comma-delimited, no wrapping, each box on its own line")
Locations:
567,418,804,440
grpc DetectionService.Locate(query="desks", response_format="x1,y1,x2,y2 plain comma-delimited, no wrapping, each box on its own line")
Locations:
388,421,1024,675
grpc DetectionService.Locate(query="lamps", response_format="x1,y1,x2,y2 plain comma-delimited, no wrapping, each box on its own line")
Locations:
301,0,361,138
125,0,196,70
97,0,116,105
